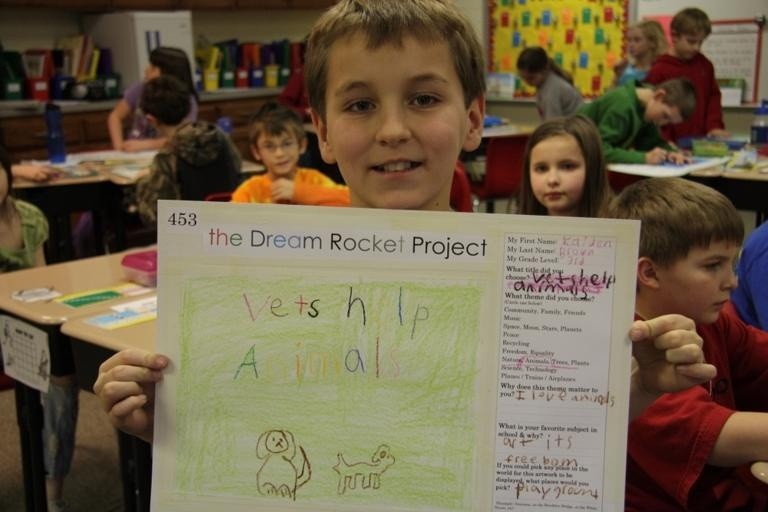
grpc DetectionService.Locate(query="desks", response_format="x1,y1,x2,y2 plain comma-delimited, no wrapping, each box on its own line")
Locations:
87,145,266,186
690,141,768,226
60,291,159,511
11,153,109,257
475,118,548,144
0,248,157,511
606,126,751,193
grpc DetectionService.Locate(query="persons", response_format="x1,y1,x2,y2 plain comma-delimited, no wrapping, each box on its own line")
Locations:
109,47,199,152
232,104,350,206
94,1,718,444
518,114,613,217
0,152,79,512
515,46,585,119
643,8,732,143
577,77,696,164
618,21,665,84
135,75,240,224
607,177,768,512
731,221,768,329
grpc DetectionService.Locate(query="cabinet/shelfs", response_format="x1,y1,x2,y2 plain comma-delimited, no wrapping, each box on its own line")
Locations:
1,84,313,160
101,11,197,100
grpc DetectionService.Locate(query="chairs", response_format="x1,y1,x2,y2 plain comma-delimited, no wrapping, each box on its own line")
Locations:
470,136,529,213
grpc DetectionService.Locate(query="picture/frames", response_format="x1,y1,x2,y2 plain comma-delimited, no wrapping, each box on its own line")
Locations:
693,17,763,105
148,200,641,512
487,0,629,103
